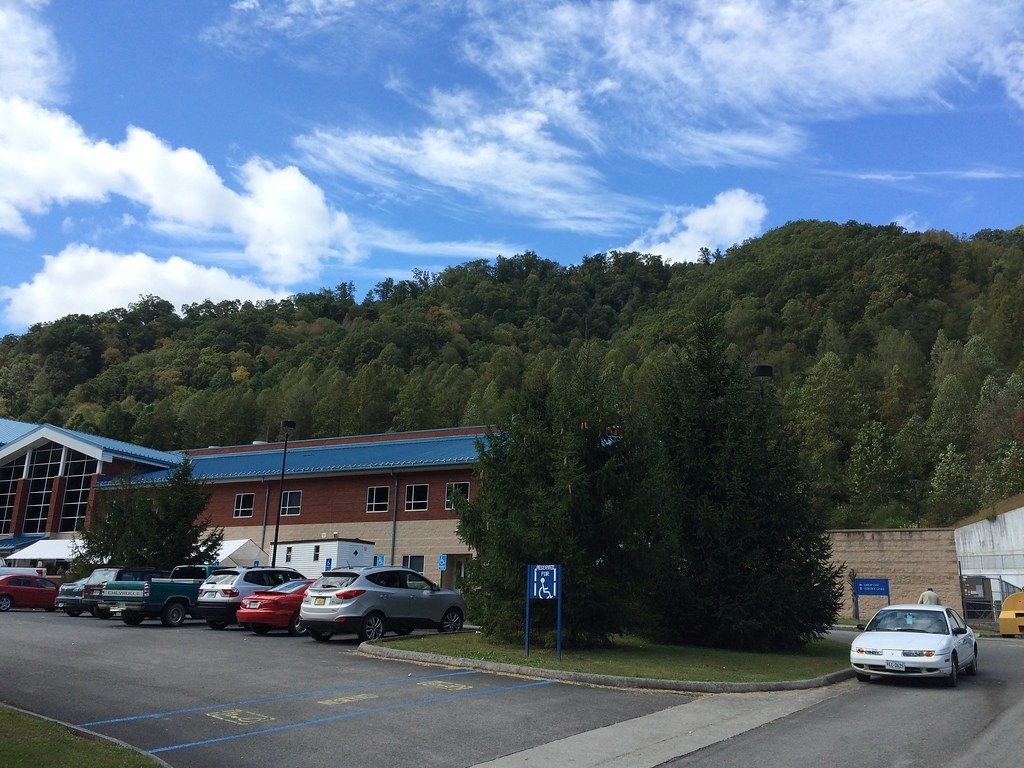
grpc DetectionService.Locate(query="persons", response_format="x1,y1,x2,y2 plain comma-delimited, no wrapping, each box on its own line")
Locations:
57,566,67,575
894,618,907,627
935,620,946,633
918,588,941,605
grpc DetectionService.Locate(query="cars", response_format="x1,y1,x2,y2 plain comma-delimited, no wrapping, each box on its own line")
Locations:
849,603,977,686
0,574,61,612
235,578,318,636
55,577,96,618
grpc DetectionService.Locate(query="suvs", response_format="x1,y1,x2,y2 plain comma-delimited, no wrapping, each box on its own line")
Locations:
298,564,469,642
194,566,309,630
82,566,173,619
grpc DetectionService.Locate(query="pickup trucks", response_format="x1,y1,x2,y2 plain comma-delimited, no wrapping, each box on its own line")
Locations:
100,564,237,628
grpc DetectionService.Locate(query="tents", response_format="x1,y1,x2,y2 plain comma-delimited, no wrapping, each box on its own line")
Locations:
6,538,269,568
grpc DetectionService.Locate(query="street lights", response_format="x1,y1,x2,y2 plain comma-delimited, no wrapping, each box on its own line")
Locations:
271,418,298,569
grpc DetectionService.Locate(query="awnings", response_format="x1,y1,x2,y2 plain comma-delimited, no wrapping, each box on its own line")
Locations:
0,537,50,550
